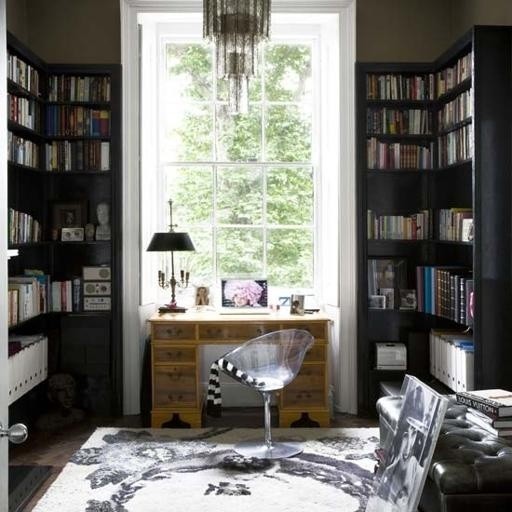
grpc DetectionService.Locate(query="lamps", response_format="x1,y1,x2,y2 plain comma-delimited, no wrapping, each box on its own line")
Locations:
146,199,197,314
202,1,272,118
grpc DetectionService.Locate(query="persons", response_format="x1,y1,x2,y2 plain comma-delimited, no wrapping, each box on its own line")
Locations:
290,300,302,315
231,293,252,308
375,385,427,508
195,287,209,305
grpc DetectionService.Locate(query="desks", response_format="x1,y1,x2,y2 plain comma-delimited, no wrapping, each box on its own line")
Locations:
145,308,331,429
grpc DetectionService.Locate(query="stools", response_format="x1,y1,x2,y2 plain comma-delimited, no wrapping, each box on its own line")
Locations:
375,394,512,511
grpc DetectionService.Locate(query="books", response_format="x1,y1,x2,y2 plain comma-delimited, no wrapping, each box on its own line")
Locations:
454,386,512,440
363,372,450,512
5,50,113,362
364,50,478,355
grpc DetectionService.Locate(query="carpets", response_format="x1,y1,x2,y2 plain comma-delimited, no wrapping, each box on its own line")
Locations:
31,427,380,511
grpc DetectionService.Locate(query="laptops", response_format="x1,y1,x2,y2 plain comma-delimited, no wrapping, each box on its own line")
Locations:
220,278,270,315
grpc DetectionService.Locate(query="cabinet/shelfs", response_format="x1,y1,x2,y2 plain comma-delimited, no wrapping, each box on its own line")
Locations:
6,31,123,446
279,321,330,428
198,323,282,345
355,26,512,420
151,323,200,428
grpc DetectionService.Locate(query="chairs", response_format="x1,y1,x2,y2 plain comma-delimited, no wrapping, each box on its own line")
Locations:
218,329,317,462
